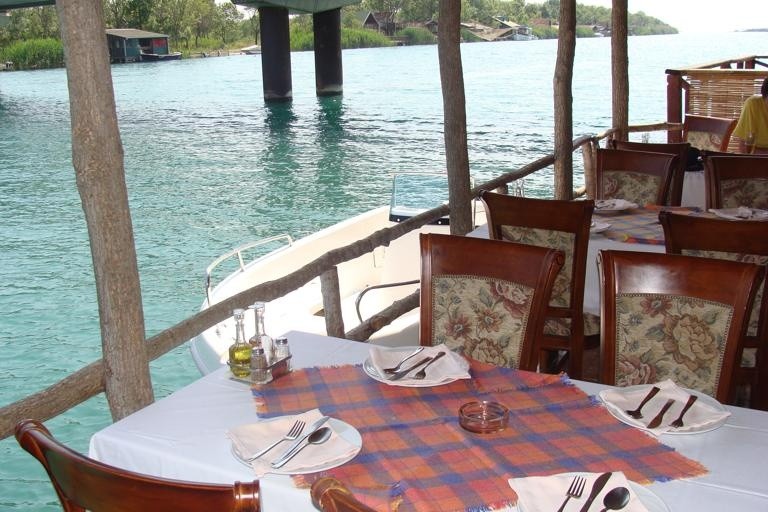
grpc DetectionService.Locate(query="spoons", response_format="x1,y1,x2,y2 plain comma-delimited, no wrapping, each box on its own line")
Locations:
384,346,422,373
272,427,333,470
624,386,662,420
597,485,631,512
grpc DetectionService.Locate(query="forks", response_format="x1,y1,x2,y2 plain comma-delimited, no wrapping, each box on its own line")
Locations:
411,350,447,381
669,395,698,430
556,474,587,512
246,420,305,463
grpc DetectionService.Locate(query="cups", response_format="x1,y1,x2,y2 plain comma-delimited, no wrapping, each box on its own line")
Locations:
513,178,525,198
642,132,650,144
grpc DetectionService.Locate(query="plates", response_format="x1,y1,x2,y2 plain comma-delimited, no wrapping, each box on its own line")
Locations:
589,198,634,214
233,416,362,476
363,350,458,388
608,386,728,437
588,218,612,233
719,206,768,221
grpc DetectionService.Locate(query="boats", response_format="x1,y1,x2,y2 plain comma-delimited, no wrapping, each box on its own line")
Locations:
513,26,536,42
240,44,261,54
139,52,181,61
187,169,498,378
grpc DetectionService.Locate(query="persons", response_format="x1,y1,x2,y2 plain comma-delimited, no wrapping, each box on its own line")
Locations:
733,75,768,154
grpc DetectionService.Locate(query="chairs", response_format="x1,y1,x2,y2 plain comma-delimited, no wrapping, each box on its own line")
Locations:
12,413,262,511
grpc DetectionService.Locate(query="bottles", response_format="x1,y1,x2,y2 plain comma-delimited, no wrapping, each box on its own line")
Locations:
275,336,291,375
249,348,267,381
228,307,253,378
249,300,274,368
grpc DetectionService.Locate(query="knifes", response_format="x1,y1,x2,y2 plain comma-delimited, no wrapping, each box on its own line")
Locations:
580,471,613,512
646,398,676,430
387,356,431,382
270,414,330,465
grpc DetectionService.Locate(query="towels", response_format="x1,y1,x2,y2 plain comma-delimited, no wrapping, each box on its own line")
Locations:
358,334,475,391
504,466,650,511
598,374,736,441
223,405,358,481
